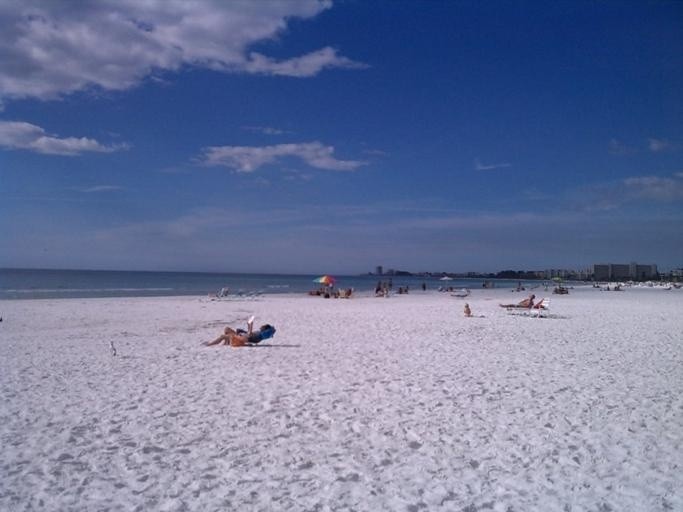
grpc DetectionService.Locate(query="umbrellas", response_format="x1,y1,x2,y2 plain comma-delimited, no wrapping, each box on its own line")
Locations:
312,276,336,283
440,277,452,280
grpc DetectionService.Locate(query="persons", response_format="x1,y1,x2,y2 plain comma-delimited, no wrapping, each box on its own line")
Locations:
375,281,426,294
309,284,354,299
438,285,453,293
497,281,568,308
207,322,271,345
593,281,624,291
464,303,472,315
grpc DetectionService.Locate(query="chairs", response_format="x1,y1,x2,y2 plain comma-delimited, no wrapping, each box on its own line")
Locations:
507,299,539,315
531,298,552,318
236,326,276,345
